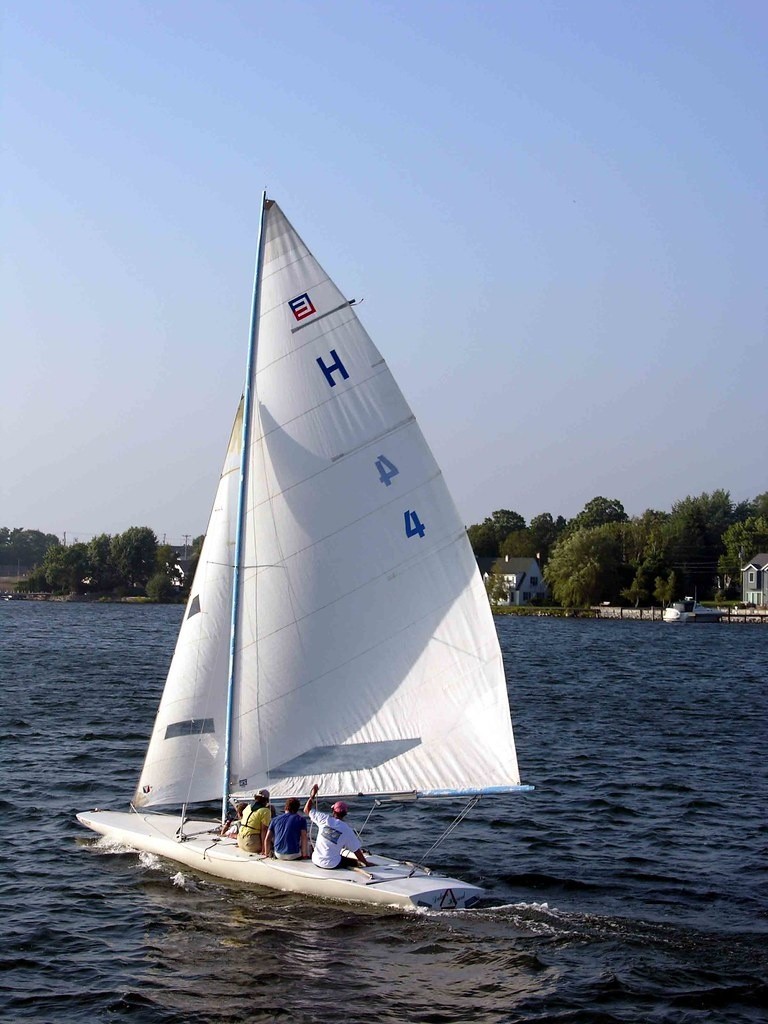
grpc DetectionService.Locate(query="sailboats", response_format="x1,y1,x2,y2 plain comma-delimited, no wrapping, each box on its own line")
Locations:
75,189,537,919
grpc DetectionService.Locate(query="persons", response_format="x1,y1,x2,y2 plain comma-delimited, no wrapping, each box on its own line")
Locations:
237,789,271,853
263,798,308,861
221,803,277,839
302,784,373,870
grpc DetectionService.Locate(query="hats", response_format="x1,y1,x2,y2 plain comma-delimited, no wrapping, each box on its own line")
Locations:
255,789,269,798
331,801,348,813
